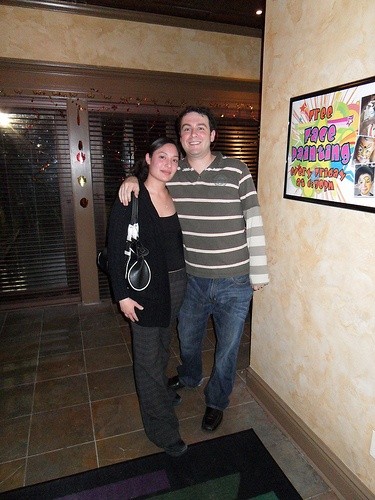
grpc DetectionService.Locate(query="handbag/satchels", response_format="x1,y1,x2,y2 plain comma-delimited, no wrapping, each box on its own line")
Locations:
95,191,153,292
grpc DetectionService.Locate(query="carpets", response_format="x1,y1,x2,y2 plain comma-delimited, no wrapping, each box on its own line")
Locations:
0,427,304,500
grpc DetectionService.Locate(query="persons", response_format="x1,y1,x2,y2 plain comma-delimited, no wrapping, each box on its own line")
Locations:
355,166,374,197
115,106,272,433
354,137,374,165
106,134,191,459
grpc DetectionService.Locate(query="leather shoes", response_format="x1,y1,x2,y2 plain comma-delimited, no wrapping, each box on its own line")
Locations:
168,375,201,390
201,405,223,433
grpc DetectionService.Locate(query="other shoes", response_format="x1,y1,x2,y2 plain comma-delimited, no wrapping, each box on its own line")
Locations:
154,438,187,457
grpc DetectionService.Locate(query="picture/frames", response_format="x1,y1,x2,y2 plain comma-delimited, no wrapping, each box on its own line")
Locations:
282,76,375,214
